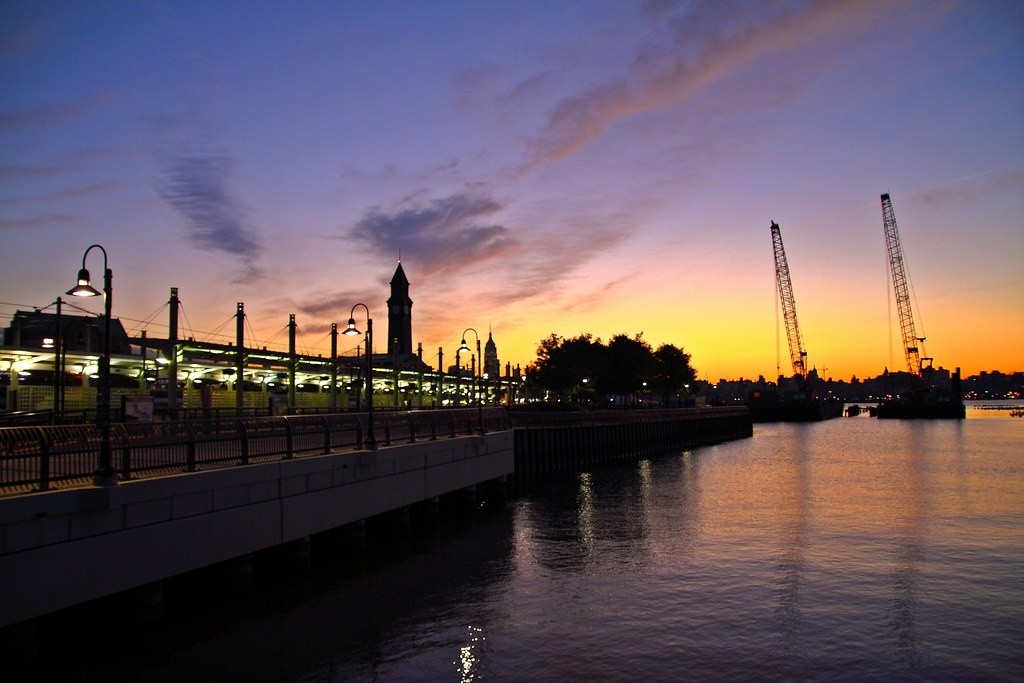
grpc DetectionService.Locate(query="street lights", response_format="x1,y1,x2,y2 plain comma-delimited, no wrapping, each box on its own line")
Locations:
341,303,380,450
65,245,118,487
457,327,486,436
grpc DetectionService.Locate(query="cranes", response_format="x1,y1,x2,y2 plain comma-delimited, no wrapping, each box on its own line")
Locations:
879,193,934,384
769,219,810,385
